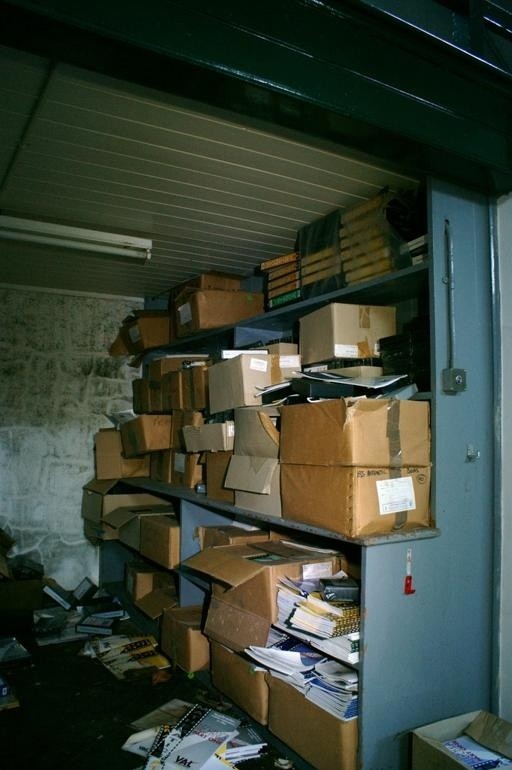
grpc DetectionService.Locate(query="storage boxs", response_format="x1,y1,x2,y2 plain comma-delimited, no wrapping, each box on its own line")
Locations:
410,708,512,770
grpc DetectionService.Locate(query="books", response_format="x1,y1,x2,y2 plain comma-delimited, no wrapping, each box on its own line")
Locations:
212,350,271,362
152,354,209,361
289,369,409,389
126,554,361,770
